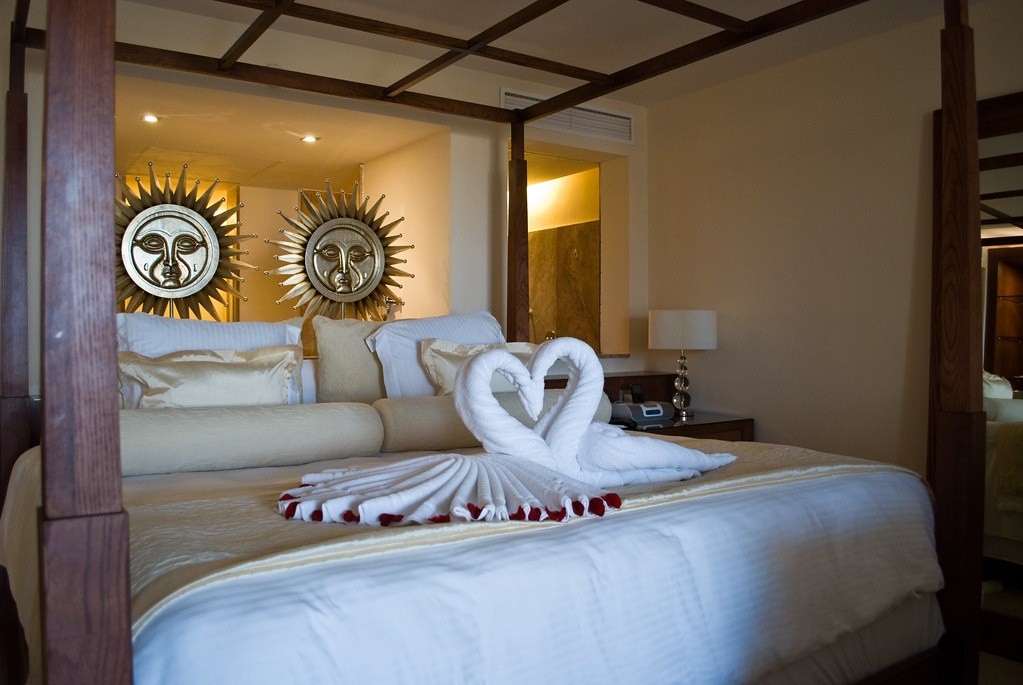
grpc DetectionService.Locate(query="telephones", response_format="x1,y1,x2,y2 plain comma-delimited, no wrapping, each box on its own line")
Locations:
630,383,645,404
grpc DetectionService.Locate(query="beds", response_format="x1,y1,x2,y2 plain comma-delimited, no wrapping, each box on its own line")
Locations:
983,421,1023,565
0,359,945,685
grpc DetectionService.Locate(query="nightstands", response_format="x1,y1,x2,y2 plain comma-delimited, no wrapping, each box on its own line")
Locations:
628,408,755,443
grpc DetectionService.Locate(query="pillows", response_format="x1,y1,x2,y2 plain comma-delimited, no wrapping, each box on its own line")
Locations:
117,344,303,408
374,388,564,452
982,369,1013,399
419,337,537,396
119,402,383,477
117,312,305,409
983,398,1023,421
366,310,507,398
312,315,387,404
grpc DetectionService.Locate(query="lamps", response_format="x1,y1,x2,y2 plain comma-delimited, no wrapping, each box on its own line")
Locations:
646,309,718,418
